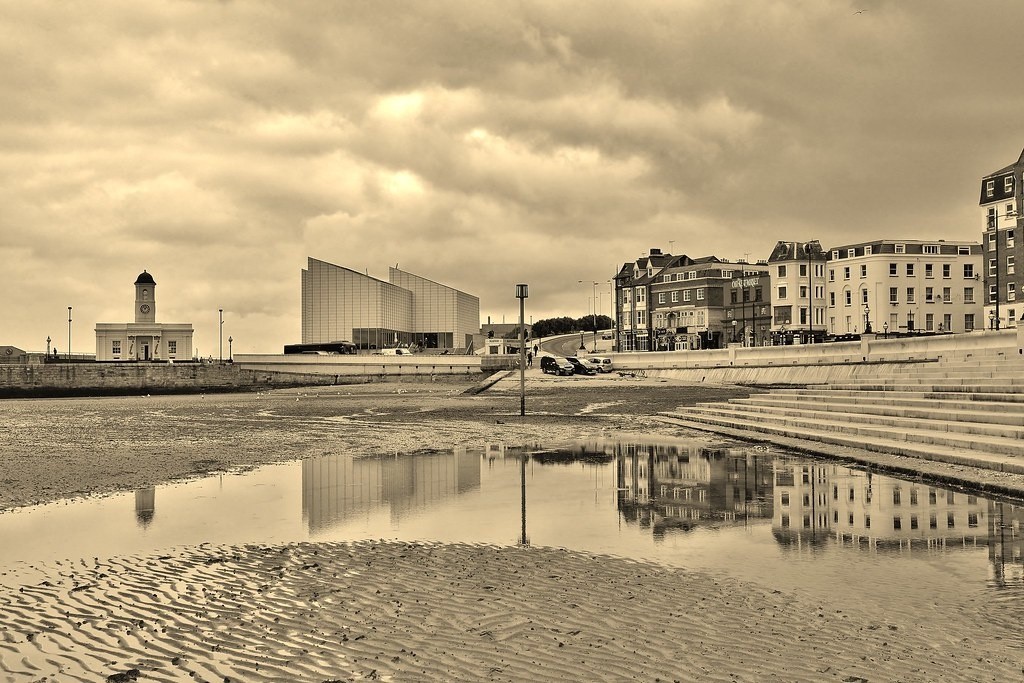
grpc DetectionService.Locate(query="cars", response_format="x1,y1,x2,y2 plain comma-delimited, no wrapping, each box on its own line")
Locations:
601,331,614,339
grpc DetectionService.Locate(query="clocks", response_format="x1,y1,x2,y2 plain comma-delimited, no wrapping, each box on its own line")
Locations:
139,304,151,313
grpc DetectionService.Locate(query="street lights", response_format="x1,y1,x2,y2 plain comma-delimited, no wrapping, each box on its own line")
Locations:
219,309,226,362
579,278,615,351
995,206,1019,329
515,283,529,413
228,336,233,360
807,248,827,343
741,263,751,346
67,305,73,361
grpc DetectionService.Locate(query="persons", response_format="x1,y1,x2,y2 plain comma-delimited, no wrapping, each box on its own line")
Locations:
528,352,533,367
53,347,57,356
208,355,213,363
487,329,494,339
200,357,204,363
522,328,528,339
534,344,538,357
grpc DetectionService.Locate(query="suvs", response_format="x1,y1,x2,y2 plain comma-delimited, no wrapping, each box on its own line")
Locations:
540,355,613,376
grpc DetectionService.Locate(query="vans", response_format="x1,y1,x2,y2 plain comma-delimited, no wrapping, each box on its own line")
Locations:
381,347,414,356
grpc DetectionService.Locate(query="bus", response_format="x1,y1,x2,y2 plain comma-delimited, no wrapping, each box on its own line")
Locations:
285,339,358,356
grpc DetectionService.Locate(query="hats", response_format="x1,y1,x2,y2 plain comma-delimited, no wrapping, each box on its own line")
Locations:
420,340,422,341
425,338,427,339
